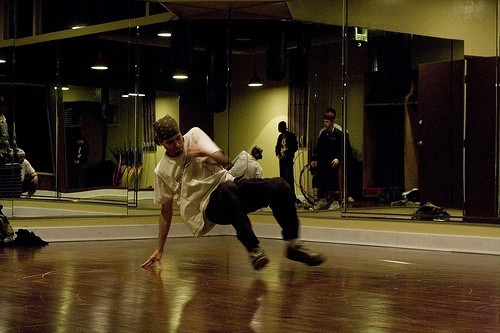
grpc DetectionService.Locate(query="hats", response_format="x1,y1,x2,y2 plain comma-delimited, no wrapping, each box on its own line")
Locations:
278,121,289,130
16,148,26,157
152,114,180,140
323,112,335,123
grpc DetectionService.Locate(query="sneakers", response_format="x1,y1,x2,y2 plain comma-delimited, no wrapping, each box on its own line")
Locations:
314,198,328,209
328,200,340,210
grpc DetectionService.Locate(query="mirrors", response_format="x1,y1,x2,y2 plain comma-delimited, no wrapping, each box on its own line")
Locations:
1,1,466,219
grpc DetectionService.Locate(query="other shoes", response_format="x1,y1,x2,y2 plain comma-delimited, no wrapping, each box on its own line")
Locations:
287,242,326,265
249,246,270,270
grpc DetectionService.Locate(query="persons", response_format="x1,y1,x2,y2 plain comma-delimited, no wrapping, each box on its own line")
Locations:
229,145,264,179
141,114,326,271
310,106,354,211
71,135,88,190
15,148,38,198
275,120,298,200
